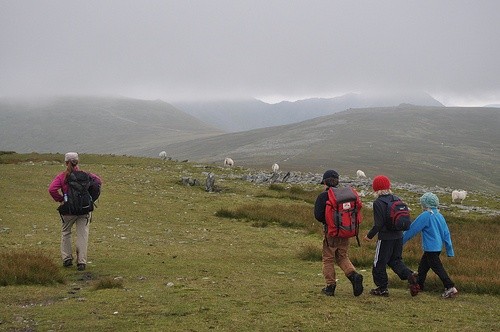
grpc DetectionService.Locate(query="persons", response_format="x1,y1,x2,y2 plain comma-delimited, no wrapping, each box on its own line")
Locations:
48,152,101,272
314,170,363,296
403,192,458,298
363,176,420,297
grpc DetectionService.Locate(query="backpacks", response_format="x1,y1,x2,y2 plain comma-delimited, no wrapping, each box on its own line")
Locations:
379,195,410,232
66,170,93,215
325,187,361,238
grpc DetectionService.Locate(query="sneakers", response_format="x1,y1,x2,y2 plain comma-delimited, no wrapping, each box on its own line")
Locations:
441,286,458,298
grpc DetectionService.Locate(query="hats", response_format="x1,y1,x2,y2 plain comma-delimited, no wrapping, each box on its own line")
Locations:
373,175,390,192
320,170,338,184
65,152,79,161
420,191,439,209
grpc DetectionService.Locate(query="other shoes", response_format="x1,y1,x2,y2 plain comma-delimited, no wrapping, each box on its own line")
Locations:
351,273,363,297
322,284,336,296
77,264,85,270
64,259,73,267
370,287,388,297
407,273,420,297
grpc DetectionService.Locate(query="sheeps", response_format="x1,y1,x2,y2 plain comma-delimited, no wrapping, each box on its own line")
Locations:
452,190,467,203
224,157,233,167
272,164,279,171
356,170,366,179
158,152,168,160
